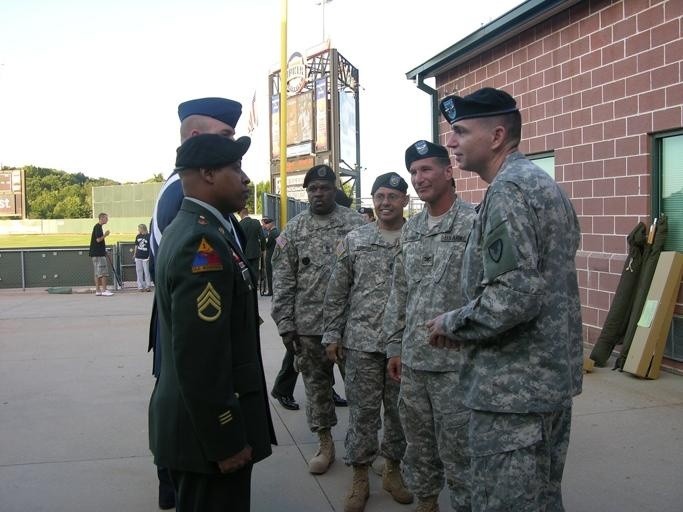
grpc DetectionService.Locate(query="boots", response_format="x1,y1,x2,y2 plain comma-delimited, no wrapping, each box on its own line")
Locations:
343,463,371,512
307,425,337,476
380,454,415,505
368,438,393,477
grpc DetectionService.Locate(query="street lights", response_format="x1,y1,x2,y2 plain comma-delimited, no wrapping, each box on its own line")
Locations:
316,0,332,40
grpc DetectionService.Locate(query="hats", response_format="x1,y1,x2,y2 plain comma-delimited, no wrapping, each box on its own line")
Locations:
174,132,252,169
303,164,336,189
176,96,244,130
438,85,519,123
404,139,449,174
370,171,409,196
263,219,274,224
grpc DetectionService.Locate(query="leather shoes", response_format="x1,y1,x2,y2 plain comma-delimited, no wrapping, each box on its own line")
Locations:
330,388,349,409
263,292,273,296
268,390,301,409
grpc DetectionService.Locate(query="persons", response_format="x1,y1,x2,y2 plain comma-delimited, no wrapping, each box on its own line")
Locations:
146,95,243,382
320,171,415,512
147,132,277,511
133,224,151,292
237,207,277,295
271,188,353,411
357,206,373,222
382,139,477,512
89,212,115,296
426,85,581,512
269,163,366,475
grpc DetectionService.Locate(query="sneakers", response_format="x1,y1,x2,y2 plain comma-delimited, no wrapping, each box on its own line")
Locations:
94,290,114,297
